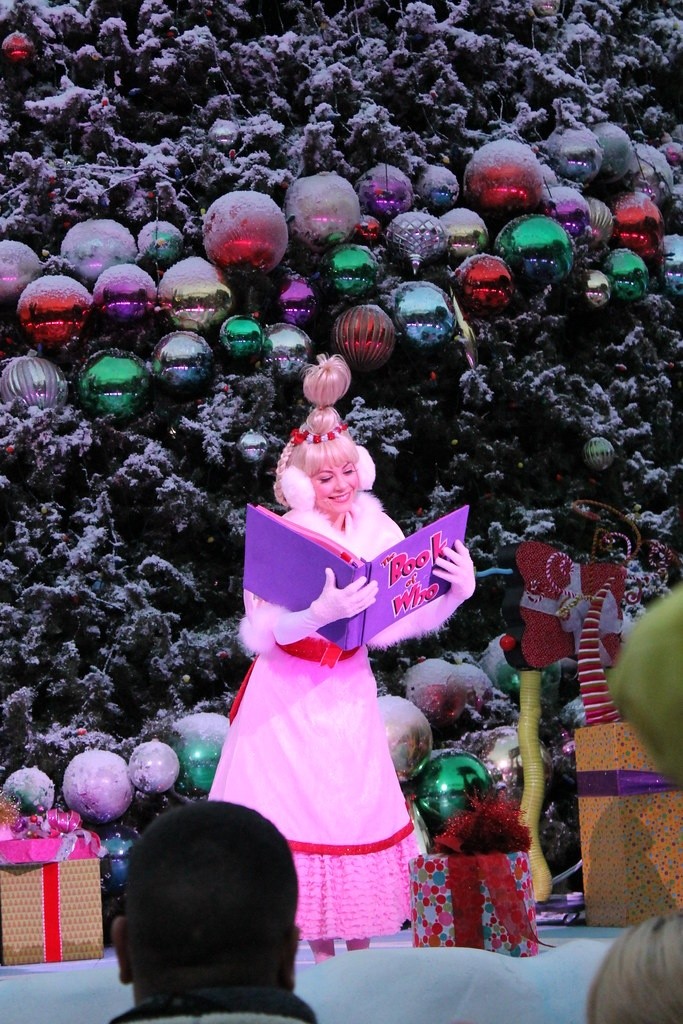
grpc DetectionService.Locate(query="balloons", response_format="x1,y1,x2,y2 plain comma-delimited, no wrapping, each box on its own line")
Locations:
94,829,140,896
404,633,585,867
4,769,54,817
0,33,36,61
129,739,181,794
0,127,683,416
412,750,494,834
376,694,434,783
64,751,132,821
170,713,231,793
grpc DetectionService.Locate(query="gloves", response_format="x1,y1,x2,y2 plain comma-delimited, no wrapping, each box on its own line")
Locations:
431,538,478,622
268,566,378,646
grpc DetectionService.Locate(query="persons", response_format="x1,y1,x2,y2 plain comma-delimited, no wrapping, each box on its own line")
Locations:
586,583,683,1024
211,353,476,961
99,801,322,1023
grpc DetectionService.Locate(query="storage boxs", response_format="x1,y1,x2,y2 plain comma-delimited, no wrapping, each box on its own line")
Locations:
573,720,683,929
0,857,104,966
0,838,100,865
0,815,43,842
407,853,538,958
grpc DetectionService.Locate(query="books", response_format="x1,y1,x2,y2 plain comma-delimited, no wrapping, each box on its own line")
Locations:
242,502,469,650
